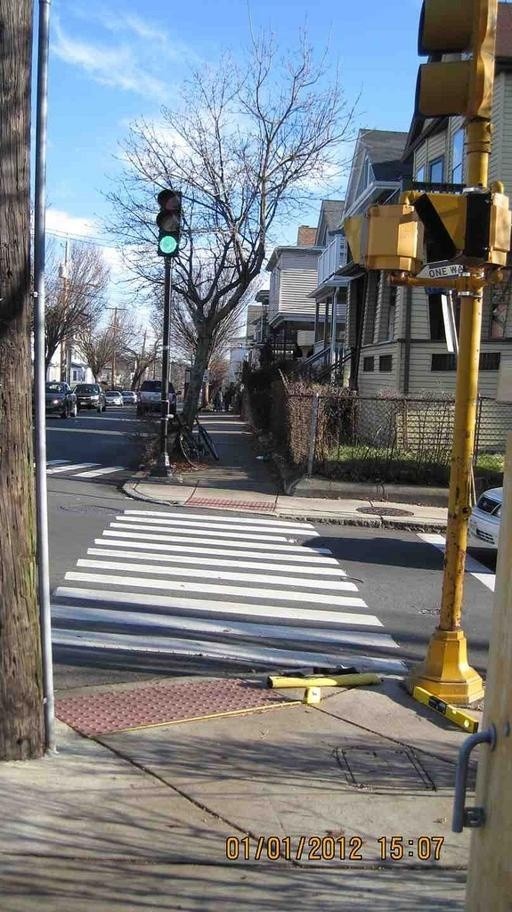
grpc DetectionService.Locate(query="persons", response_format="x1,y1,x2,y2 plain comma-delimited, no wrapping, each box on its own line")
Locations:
215,386,224,414
215,392,221,413
225,385,230,413
99,380,110,393
225,381,236,410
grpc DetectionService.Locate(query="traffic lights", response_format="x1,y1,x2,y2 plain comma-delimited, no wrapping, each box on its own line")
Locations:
418,1,502,125
340,203,419,274
155,189,183,259
463,181,510,271
414,190,465,260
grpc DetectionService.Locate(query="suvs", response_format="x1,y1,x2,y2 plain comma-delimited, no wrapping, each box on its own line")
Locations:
138,380,182,414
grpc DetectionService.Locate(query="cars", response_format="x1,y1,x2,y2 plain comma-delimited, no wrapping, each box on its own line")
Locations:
470,489,504,549
31,382,137,417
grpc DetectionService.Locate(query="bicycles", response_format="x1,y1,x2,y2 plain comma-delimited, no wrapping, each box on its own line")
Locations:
174,404,221,466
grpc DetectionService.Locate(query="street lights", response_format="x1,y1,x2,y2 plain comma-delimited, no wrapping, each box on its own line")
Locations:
58,241,71,382
